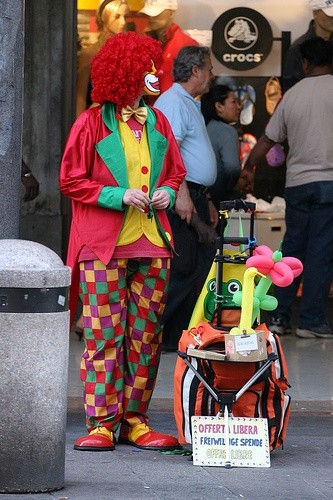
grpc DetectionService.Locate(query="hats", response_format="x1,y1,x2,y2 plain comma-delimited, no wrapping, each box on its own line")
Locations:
137,0,178,17
310,0,333,17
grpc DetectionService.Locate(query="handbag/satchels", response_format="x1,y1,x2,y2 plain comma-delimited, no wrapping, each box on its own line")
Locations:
188,207,247,330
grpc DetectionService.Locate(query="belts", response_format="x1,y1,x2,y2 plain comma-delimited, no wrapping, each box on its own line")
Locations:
186,180,209,195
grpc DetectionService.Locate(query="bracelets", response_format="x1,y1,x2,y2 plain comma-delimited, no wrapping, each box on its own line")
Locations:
207,197,213,202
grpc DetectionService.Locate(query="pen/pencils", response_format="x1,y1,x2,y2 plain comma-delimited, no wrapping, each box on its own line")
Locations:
189,332,202,346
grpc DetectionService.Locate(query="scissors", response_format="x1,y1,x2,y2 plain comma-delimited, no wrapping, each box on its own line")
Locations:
190,325,205,344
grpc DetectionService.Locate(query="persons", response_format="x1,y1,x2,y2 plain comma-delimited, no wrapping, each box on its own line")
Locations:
151,45,241,354
137,0,198,107
76,0,129,118
282,0,333,94
241,35,333,339
59,33,187,451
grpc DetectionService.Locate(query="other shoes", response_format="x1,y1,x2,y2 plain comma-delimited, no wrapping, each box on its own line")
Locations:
265,143,285,168
268,315,293,336
295,320,333,339
264,75,283,116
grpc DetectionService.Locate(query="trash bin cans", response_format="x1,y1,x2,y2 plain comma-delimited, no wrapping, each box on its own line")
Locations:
0,239,72,494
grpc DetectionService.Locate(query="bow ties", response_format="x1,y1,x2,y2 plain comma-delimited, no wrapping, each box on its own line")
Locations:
121,104,149,125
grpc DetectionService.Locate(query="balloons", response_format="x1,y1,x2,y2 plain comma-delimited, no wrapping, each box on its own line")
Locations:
229,245,303,336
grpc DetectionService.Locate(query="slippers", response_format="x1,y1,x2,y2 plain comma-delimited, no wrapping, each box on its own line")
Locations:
239,84,256,126
239,134,258,175
226,83,239,125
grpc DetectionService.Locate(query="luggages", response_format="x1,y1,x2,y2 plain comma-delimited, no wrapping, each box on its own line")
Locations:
171,198,292,455
